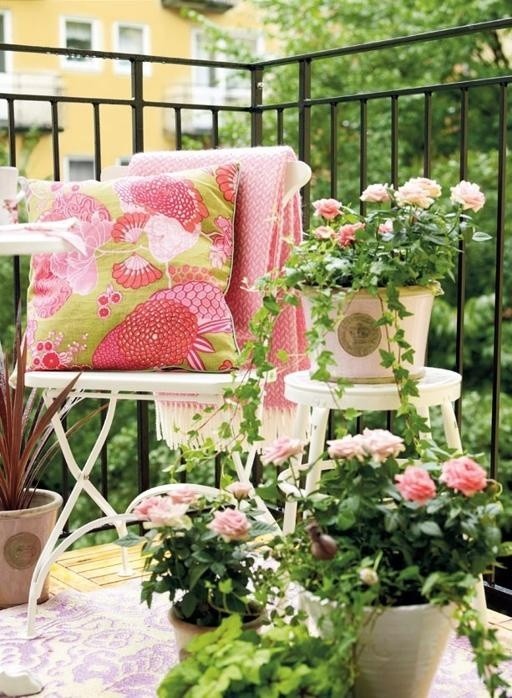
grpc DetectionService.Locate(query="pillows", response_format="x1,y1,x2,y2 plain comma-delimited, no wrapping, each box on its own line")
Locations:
21,162,246,374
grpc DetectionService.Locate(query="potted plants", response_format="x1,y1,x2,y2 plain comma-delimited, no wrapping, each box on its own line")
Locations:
0,332,111,612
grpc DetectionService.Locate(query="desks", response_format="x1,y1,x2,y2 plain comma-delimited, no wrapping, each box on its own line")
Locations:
0,226,71,257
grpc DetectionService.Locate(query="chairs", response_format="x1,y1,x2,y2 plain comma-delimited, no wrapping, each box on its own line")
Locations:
24,158,312,617
